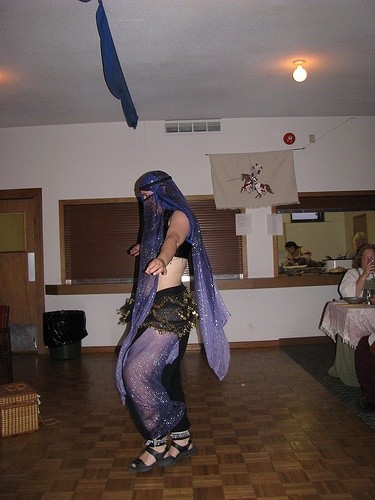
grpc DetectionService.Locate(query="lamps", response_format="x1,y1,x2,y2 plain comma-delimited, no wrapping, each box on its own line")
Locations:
292,60,307,82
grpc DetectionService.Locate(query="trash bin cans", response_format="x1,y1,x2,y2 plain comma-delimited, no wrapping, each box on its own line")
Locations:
43,310,88,361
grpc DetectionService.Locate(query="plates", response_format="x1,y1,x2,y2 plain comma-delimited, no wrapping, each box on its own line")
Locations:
343,297,366,304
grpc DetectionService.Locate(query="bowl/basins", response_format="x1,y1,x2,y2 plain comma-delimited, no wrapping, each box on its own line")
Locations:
283,267,302,275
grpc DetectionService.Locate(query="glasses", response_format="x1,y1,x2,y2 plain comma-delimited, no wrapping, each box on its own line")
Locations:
361,257,375,262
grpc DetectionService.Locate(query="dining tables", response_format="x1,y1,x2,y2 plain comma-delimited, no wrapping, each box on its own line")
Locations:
319,301,375,387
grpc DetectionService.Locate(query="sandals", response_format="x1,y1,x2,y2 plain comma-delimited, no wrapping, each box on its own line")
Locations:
129,439,172,472
162,437,198,465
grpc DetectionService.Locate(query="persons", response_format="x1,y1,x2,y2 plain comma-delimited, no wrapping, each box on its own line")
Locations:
347,231,369,258
115,171,231,473
285,241,312,266
338,243,375,300
355,332,375,414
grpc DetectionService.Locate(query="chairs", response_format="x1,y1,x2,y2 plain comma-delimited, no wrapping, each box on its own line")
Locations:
0,305,13,382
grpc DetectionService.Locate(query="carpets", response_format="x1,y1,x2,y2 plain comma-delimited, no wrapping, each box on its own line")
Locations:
280,343,375,429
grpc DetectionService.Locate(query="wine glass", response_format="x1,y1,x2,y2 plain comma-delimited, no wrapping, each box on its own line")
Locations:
369,259,375,279
